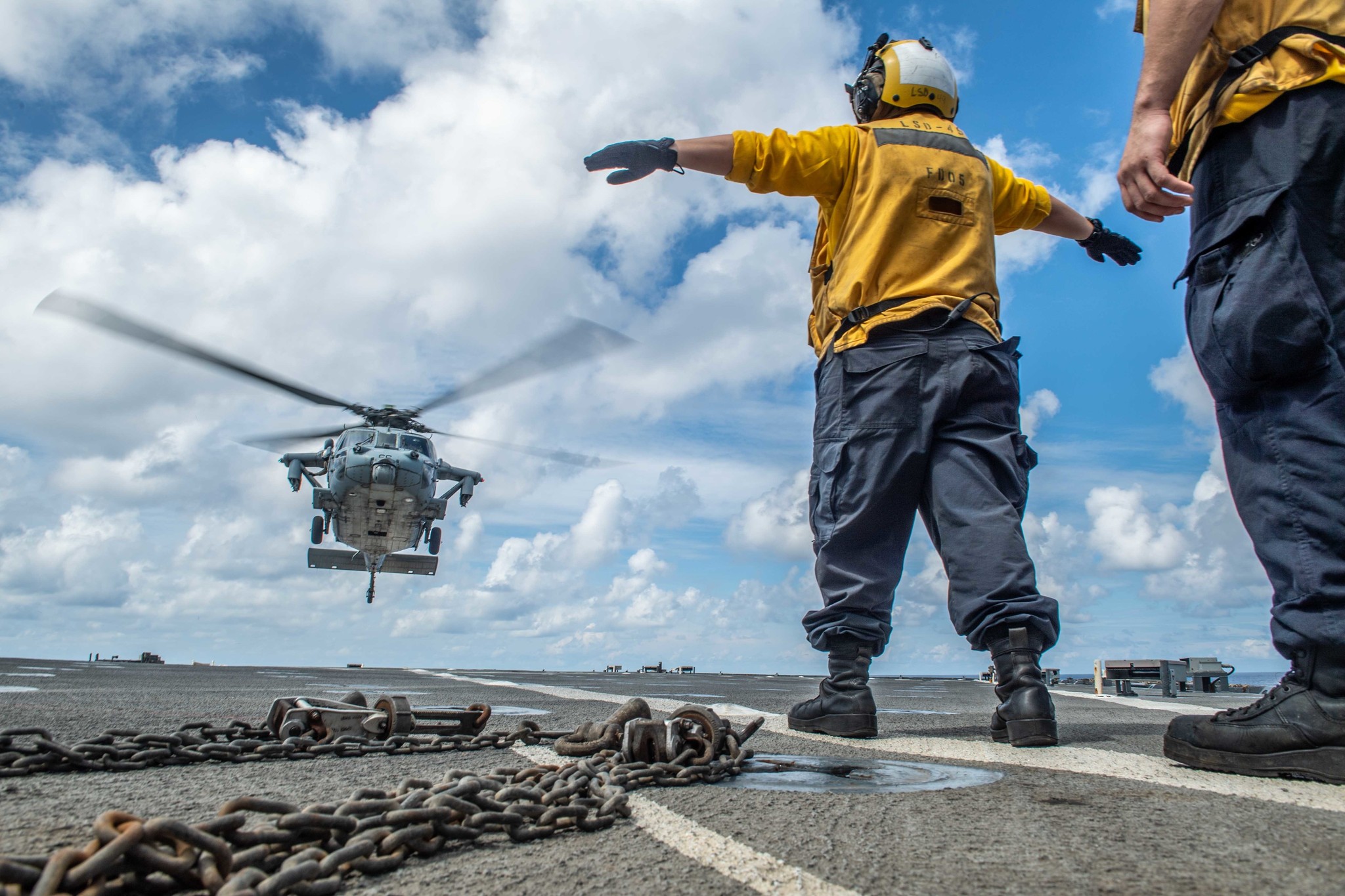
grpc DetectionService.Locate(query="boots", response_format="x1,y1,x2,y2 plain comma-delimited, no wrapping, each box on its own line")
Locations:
982,619,1060,747
1163,639,1345,787
787,635,878,738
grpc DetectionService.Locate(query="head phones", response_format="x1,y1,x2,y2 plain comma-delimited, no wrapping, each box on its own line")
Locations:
844,33,960,124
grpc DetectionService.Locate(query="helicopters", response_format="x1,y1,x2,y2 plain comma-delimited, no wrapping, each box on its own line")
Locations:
34,291,634,601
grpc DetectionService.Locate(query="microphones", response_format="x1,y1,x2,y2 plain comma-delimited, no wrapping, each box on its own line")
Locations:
863,33,890,71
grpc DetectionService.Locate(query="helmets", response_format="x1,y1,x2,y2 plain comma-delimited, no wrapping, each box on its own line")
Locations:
845,32,959,125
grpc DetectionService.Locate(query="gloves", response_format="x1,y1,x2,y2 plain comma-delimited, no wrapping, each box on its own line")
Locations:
1075,216,1143,268
584,137,684,186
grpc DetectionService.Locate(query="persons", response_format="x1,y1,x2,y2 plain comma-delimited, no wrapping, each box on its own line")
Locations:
410,439,424,453
1116,0,1345,786
583,33,1143,747
